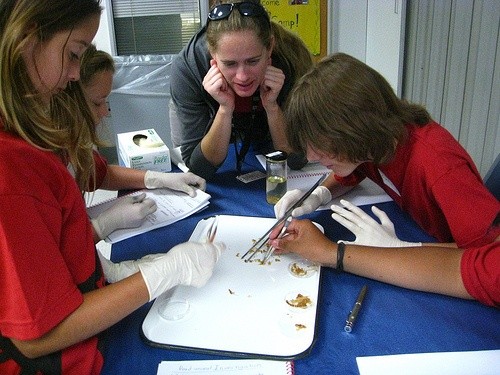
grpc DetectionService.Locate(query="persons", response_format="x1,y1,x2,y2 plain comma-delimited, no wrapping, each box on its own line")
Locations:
169,0,313,179
56,44,206,246
0,0,218,375
267,219,500,306
275,52,500,248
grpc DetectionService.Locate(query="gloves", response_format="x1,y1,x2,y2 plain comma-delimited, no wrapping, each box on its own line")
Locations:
145,170,207,198
91,194,158,239
274,186,333,217
97,250,165,284
330,199,424,249
136,241,226,303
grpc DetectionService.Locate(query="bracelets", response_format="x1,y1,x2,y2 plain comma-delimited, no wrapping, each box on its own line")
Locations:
337,242,345,272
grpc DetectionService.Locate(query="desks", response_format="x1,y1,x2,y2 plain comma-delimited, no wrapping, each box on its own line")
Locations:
100,138,500,375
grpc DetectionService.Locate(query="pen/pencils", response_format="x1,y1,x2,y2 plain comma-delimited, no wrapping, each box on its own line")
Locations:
344,283,368,333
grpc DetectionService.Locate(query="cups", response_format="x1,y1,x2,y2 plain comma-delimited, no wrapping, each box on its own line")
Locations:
265,150,289,204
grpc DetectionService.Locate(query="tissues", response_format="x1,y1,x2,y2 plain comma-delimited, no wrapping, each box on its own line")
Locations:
116,127,172,173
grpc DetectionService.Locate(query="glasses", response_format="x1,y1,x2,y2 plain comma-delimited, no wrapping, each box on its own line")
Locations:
205,0,272,31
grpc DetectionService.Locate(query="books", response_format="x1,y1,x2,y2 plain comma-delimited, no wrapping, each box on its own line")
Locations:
86,185,212,244
157,359,296,375
